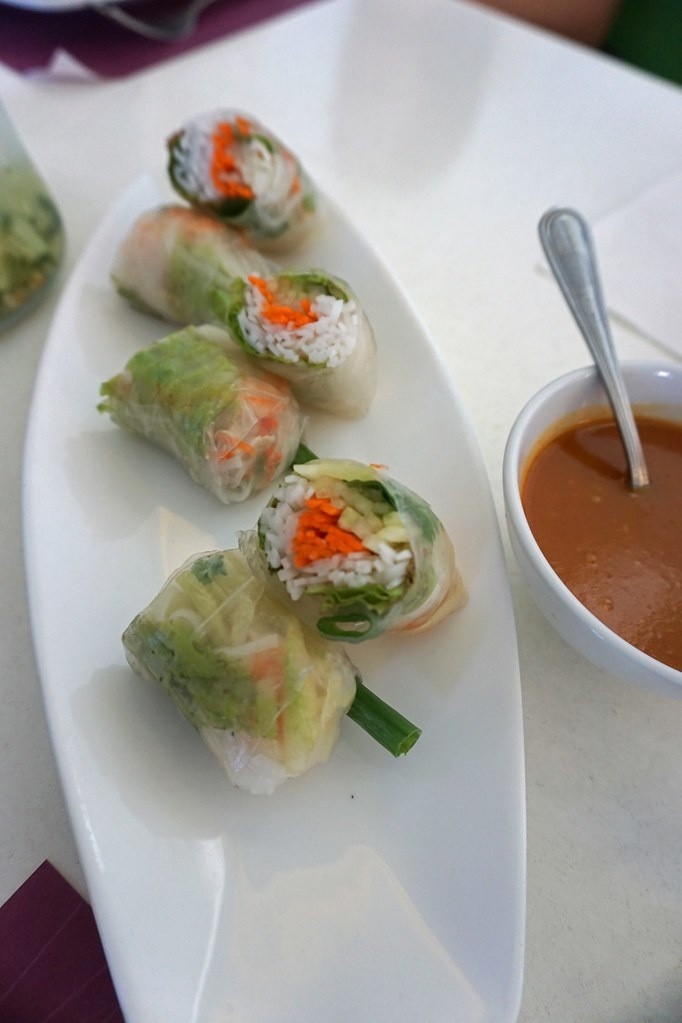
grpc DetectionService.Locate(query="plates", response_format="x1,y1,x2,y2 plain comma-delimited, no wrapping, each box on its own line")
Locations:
20,104,526,1023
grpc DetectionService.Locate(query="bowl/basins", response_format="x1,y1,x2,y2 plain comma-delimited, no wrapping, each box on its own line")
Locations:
502,356,681,694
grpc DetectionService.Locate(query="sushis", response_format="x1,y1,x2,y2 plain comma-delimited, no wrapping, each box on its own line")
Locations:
99,106,465,797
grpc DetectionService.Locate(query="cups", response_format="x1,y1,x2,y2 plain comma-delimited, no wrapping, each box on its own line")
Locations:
0,97,66,340
